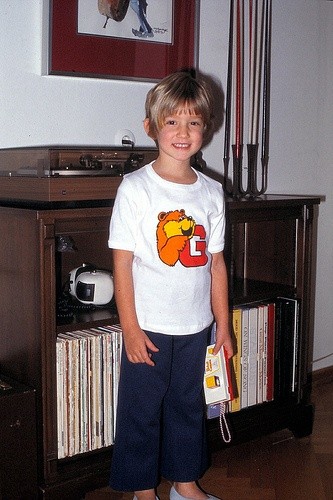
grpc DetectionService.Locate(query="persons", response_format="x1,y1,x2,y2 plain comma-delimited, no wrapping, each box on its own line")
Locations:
107,69,237,500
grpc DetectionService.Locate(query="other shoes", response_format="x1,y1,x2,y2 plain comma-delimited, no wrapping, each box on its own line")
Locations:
170,486,221,500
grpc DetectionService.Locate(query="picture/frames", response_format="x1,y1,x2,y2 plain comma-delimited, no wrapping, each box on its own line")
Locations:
44,0,202,84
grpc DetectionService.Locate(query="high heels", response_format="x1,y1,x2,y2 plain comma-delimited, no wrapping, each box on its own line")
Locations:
132,28,154,38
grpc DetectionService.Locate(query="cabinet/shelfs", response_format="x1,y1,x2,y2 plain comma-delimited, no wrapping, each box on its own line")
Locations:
0,193,322,500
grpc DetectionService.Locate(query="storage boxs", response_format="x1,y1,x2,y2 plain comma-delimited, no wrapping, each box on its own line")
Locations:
0,145,163,207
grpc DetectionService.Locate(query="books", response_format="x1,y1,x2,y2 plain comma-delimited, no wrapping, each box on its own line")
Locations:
51,299,277,460
